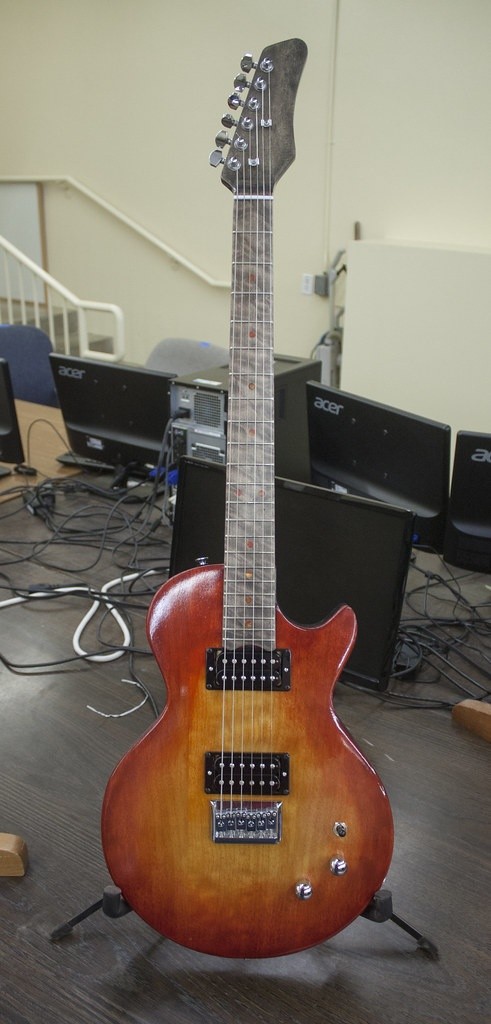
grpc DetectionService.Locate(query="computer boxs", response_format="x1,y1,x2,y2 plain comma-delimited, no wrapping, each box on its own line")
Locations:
169,354,323,483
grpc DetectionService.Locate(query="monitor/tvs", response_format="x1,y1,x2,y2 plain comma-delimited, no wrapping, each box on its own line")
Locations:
0,358,26,480
48,353,178,503
167,379,491,693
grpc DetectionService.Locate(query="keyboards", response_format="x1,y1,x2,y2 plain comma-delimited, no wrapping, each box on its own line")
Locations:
55,449,157,482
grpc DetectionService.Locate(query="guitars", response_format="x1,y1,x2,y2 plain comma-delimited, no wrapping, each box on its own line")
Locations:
101,38,395,959
